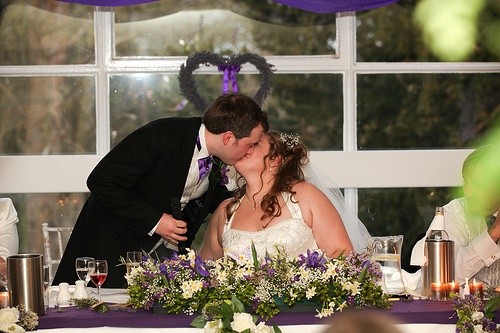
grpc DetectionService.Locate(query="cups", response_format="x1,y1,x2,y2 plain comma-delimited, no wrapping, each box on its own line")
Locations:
44,266,50,311
422,239,455,296
126,251,142,276
7,254,45,316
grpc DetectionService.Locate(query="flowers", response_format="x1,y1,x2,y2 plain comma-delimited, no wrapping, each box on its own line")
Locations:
215,158,231,185
450,291,500,333
68,247,389,333
278,131,301,148
0,303,39,333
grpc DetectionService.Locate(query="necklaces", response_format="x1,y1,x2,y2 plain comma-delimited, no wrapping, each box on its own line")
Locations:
246,199,261,210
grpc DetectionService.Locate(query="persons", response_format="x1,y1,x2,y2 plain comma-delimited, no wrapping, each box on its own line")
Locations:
52,93,270,289
197,131,370,267
425,144,500,286
0,198,20,288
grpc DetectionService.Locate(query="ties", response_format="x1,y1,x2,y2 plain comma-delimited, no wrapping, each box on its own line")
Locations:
196,135,211,178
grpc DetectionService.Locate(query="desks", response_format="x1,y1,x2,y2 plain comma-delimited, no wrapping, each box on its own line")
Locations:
38,285,456,333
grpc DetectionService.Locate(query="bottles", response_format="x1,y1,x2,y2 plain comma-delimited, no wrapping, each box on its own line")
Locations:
427,207,449,240
57,280,87,307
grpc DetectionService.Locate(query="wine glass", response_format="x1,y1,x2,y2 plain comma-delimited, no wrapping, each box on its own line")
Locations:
75,257,95,289
88,260,108,301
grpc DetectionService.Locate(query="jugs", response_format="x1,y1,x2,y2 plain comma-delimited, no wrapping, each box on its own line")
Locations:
364,235,405,296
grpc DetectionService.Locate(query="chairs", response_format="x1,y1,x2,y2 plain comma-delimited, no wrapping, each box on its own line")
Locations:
42,222,74,287
